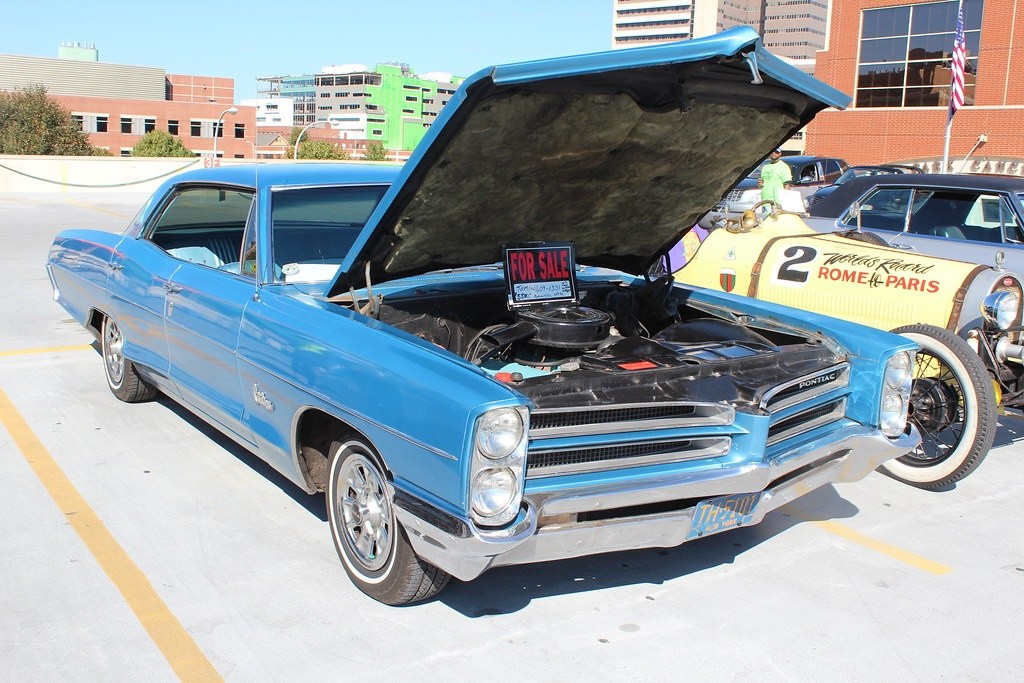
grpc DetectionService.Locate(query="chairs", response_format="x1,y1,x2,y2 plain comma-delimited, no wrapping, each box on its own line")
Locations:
927,227,967,241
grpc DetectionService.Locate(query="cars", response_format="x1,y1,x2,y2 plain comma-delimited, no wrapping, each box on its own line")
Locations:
639,196,1024,493
696,152,1024,283
45,22,926,607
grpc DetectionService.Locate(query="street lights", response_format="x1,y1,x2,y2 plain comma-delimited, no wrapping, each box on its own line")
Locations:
213,107,239,157
293,119,340,159
246,140,255,158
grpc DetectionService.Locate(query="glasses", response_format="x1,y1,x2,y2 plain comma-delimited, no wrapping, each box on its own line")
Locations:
775,151,780,154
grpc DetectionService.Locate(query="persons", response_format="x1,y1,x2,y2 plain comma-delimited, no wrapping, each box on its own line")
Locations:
759,147,792,221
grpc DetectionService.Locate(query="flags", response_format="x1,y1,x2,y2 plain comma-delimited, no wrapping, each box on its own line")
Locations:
947,3,965,124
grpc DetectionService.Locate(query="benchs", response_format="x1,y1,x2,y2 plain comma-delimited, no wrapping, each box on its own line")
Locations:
860,215,963,235
151,226,360,265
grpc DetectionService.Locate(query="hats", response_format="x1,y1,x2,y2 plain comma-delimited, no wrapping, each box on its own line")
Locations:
775,147,781,153
